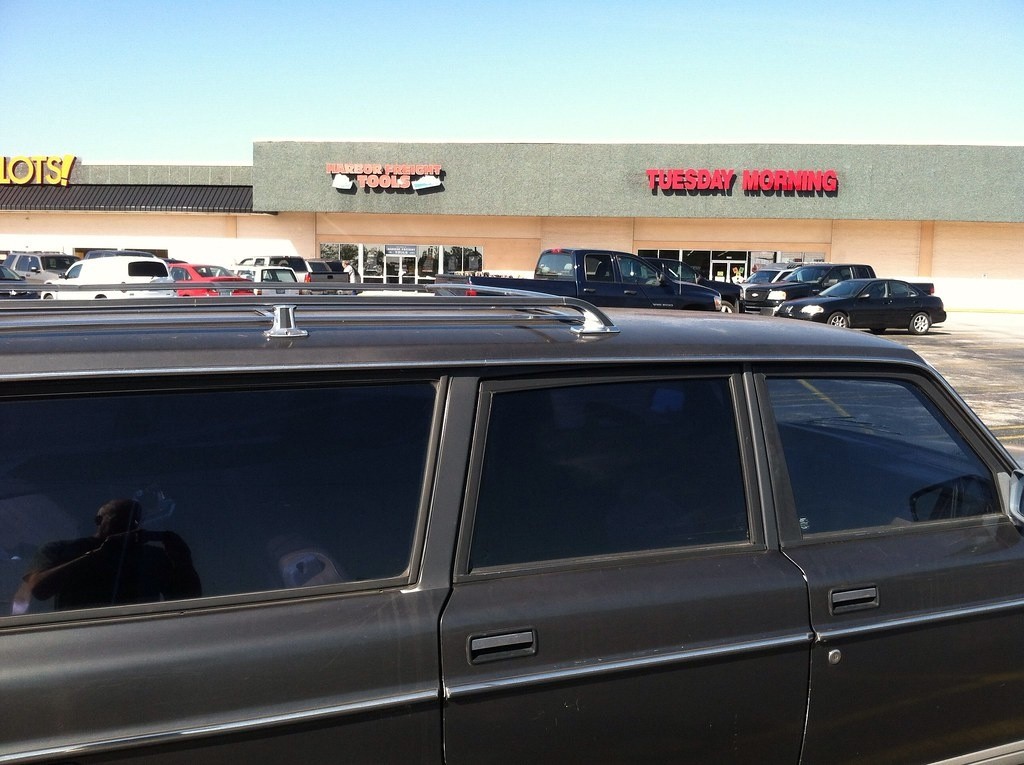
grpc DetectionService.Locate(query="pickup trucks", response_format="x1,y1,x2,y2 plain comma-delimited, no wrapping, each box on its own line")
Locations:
236,256,351,296
746,262,937,318
431,247,723,316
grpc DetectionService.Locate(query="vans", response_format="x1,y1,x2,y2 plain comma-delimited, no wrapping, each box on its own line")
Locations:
83,249,157,259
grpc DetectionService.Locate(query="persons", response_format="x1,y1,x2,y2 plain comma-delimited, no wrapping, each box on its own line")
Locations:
21,499,202,611
338,260,358,296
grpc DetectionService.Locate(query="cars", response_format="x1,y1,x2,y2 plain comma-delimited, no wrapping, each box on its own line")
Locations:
0,265,37,298
770,280,948,336
4,253,83,297
593,256,748,317
735,268,796,299
0,278,1024,764
39,255,175,300
230,267,301,297
166,263,255,297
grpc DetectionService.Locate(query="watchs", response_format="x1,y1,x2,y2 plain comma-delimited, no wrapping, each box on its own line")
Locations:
85,550,95,559
